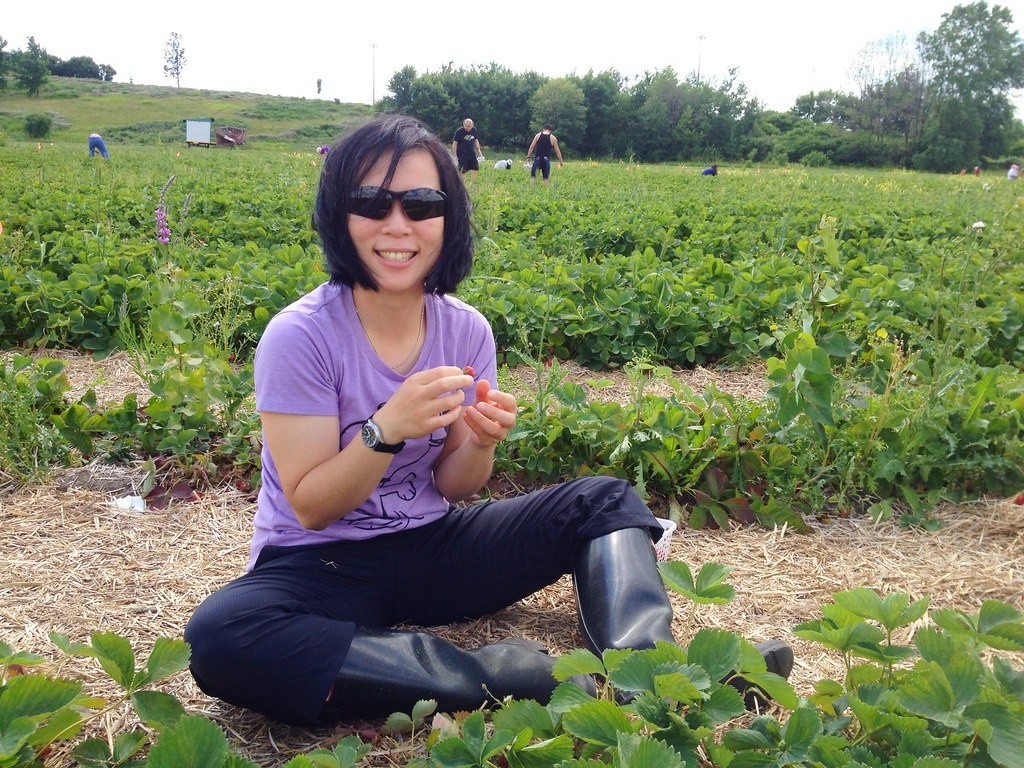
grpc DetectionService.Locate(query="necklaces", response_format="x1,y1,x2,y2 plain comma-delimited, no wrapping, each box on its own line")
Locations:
354,291,425,369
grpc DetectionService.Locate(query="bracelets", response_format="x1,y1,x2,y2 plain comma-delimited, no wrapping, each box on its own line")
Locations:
527,156,530,158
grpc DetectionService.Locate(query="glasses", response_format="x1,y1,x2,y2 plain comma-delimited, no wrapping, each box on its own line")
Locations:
347,186,447,222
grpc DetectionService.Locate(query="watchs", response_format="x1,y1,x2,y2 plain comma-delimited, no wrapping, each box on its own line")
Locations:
361,412,406,454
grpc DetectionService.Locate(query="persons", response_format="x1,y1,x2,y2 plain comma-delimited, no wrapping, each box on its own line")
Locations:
961,167,967,175
974,164,980,175
88,134,109,157
316,146,329,154
184,114,794,726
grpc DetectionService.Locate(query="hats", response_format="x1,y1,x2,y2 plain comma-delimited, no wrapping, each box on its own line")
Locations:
507,159,512,165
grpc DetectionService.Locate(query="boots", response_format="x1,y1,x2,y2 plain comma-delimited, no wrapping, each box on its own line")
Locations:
326,630,597,724
570,526,795,716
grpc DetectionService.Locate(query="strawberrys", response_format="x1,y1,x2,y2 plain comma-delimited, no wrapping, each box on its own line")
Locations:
462,366,475,377
